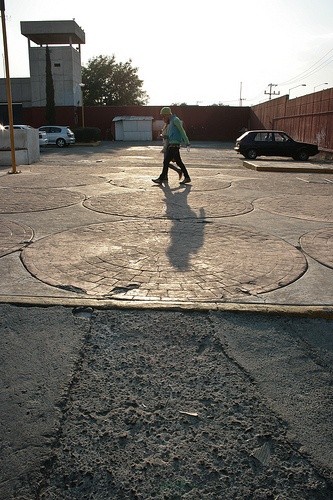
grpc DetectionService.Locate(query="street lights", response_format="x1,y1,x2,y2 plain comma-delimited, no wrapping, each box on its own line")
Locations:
314,83,328,92
288,84,307,97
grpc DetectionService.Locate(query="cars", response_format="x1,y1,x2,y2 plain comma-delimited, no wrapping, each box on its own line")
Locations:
36,125,76,148
3,124,48,147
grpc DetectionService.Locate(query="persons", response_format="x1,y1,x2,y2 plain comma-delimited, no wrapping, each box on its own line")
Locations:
151,107,191,184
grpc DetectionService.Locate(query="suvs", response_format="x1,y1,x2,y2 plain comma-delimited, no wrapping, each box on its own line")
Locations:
234,130,320,162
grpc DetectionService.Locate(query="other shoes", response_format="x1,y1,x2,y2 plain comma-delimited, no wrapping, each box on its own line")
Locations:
152,178,163,186
179,177,191,184
163,178,168,182
178,169,182,180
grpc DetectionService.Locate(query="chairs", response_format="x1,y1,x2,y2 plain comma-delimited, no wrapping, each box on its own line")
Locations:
265,136,272,141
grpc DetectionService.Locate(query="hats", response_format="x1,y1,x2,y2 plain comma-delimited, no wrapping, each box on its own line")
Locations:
160,107,172,115
